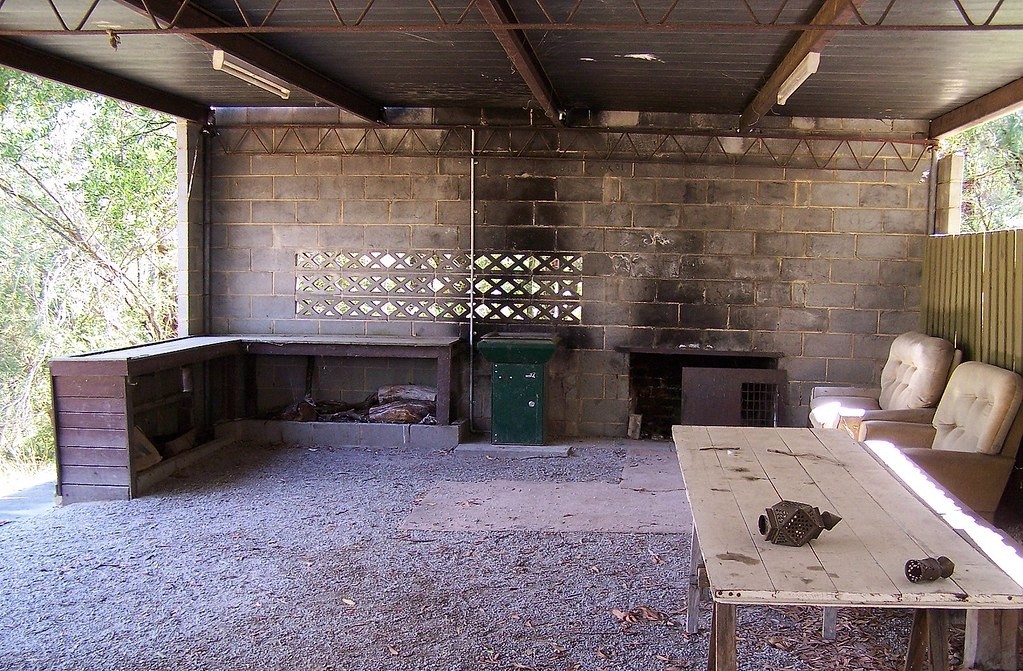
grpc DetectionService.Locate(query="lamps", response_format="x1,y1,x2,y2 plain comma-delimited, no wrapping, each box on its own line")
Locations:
777,51,821,105
212,49,291,100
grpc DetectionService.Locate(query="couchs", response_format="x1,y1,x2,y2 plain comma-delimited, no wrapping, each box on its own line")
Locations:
860,361,1023,525
806,330,962,428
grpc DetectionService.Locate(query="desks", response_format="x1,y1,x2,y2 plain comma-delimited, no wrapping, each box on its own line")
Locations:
670,423,1023,670
